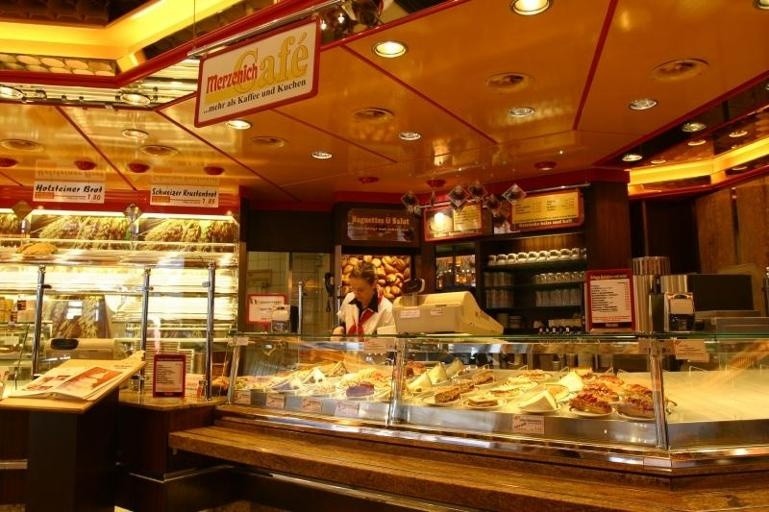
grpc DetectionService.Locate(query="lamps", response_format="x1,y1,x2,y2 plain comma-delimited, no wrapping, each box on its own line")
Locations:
401,180,592,218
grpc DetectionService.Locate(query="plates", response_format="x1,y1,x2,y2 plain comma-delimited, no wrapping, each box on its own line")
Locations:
517,403,563,413
463,382,538,411
308,387,378,399
424,394,461,407
570,406,657,422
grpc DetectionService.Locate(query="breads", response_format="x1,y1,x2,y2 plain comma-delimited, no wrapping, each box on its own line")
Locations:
271,360,666,419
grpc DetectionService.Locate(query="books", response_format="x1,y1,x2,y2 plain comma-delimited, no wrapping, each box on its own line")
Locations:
7,364,134,401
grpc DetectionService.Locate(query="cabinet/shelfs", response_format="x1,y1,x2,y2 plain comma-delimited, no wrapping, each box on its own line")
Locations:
0,209,769,512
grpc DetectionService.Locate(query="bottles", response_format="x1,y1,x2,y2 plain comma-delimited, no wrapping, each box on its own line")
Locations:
270,306,290,335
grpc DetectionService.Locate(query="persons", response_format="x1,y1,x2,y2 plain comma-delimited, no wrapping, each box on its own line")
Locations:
330,260,397,343
